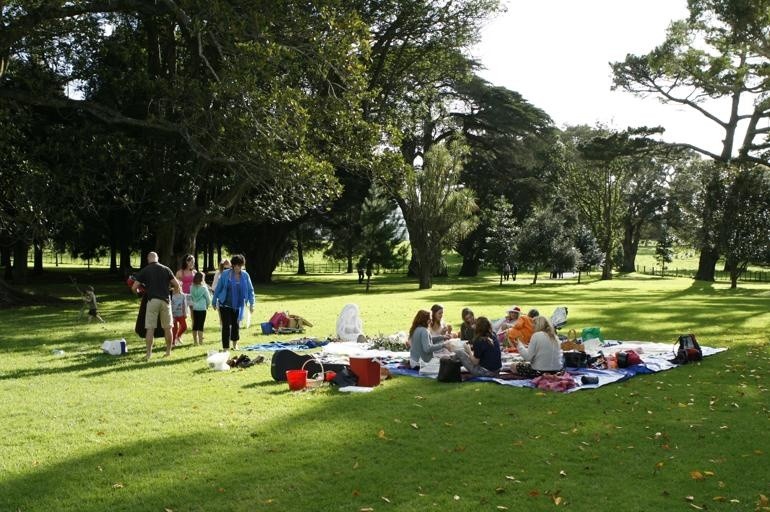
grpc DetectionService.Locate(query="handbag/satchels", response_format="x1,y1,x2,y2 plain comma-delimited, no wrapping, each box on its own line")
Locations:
563,350,643,370
435,357,463,384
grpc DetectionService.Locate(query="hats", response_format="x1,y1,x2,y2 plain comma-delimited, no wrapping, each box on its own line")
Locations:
505,306,524,313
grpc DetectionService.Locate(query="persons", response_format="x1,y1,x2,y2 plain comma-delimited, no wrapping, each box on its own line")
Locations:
130,250,257,363
406,303,564,381
81,285,106,325
548,267,553,279
511,264,518,280
356,260,366,284
558,267,564,279
502,262,511,280
553,264,558,278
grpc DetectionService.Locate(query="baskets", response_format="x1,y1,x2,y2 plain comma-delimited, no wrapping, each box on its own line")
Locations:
560,328,585,353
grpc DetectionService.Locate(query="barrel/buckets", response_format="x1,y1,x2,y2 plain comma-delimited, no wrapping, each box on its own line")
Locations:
286,369,307,389
349,355,380,386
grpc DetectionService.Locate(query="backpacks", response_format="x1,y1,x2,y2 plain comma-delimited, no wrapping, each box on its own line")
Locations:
673,333,703,364
